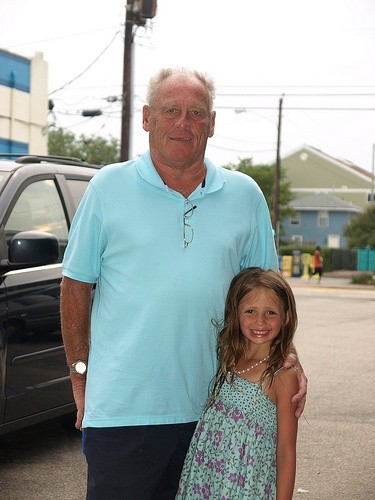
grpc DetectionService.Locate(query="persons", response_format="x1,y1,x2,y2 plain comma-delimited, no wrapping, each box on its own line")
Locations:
60,71,308,500
309,246,324,282
175,267,298,500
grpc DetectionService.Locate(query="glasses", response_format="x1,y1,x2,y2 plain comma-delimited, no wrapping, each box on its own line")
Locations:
183,198,197,249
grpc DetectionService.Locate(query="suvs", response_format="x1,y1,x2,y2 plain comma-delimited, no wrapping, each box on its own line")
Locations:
0,152,106,436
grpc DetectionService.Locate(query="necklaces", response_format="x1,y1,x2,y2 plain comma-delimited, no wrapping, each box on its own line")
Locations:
232,356,270,374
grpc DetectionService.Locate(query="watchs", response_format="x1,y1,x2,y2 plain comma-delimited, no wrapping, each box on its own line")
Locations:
69,359,88,375
